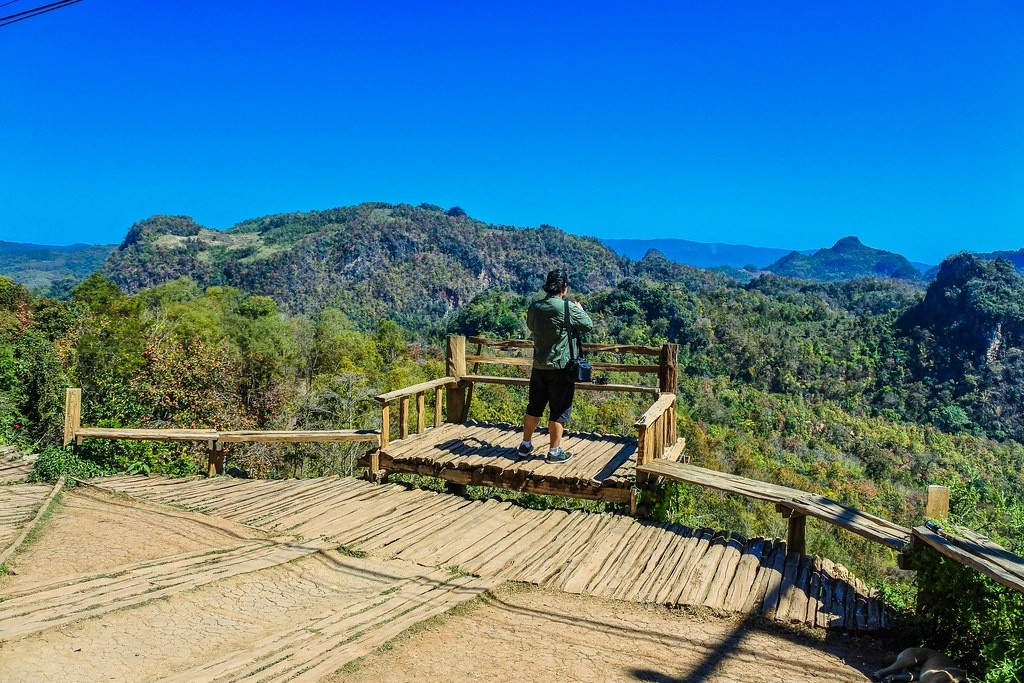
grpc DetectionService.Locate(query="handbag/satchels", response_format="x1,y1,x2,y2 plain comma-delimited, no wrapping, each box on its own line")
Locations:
563,358,592,382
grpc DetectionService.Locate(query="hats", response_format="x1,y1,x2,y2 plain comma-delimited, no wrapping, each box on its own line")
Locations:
546,269,570,286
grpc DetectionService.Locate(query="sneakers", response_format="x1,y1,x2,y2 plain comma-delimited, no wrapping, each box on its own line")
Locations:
546,447,573,464
518,443,536,457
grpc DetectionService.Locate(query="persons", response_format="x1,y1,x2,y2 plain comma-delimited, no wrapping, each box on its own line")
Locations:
519,270,594,464
